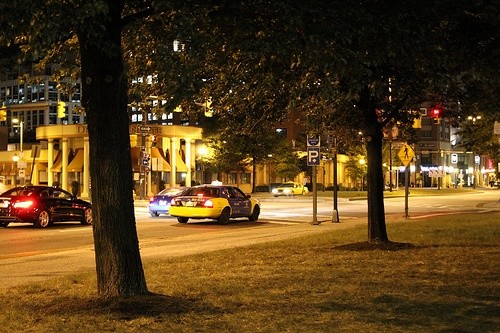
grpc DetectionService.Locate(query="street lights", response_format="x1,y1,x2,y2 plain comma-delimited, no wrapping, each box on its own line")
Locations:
198,146,207,184
468,115,482,188
13,118,23,186
12,153,19,187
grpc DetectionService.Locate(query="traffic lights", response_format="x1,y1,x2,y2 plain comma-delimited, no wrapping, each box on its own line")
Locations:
151,134,158,142
57,101,66,119
204,96,214,118
433,104,442,125
0,106,6,121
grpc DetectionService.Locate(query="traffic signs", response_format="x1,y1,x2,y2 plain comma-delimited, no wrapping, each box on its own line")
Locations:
138,126,152,133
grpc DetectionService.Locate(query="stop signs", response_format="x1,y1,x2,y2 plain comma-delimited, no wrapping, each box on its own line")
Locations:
306,134,320,147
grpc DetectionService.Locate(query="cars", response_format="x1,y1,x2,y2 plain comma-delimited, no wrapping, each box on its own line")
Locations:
168,180,261,226
148,186,192,217
0,185,93,228
271,181,309,197
